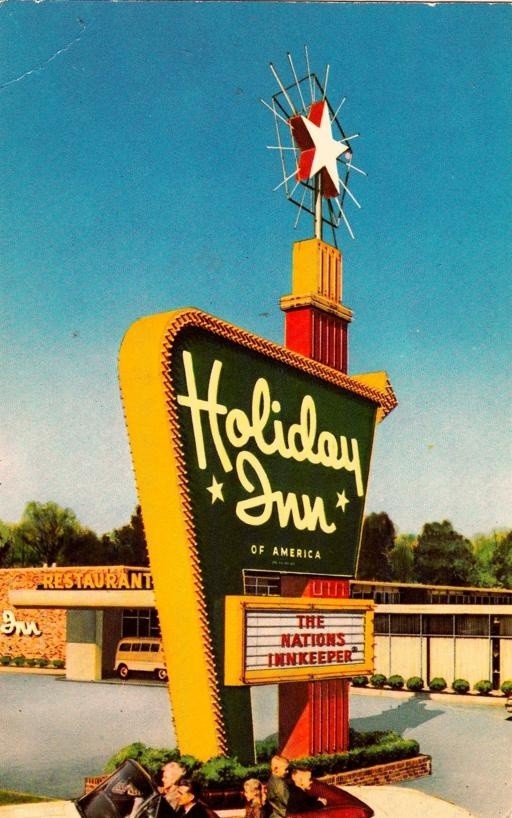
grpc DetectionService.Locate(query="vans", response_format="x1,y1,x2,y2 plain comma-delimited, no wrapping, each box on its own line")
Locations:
112,634,167,681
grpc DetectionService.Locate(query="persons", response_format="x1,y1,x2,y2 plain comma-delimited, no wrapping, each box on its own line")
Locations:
264,752,292,818
157,761,188,818
286,764,328,813
243,776,269,817
177,776,210,817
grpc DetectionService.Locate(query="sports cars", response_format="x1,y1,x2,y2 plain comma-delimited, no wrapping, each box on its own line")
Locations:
0,759,473,818
